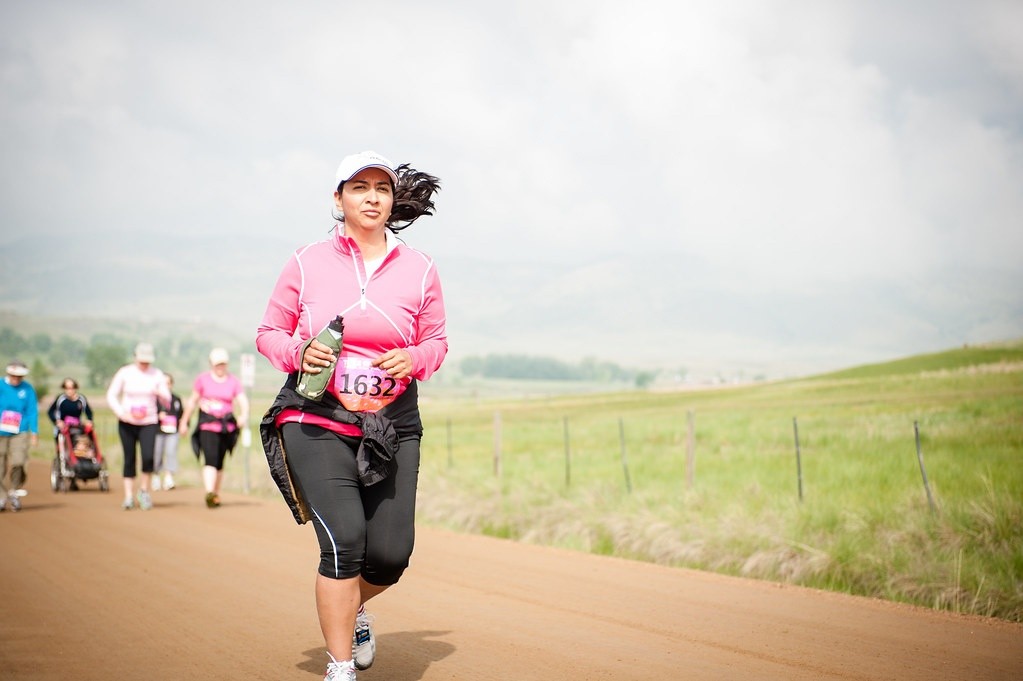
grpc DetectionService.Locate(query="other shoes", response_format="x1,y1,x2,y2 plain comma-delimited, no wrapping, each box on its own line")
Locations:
120,497,135,511
205,491,221,509
7,496,21,512
150,475,160,491
162,474,175,491
136,492,152,512
0,496,7,508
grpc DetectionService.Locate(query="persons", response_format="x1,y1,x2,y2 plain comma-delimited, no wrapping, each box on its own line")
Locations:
0,359,40,512
252,151,449,681
106,342,173,512
179,349,249,507
151,374,184,491
47,377,93,491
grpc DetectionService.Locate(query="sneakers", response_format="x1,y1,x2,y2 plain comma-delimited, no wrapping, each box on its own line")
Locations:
352,604,376,671
323,651,356,681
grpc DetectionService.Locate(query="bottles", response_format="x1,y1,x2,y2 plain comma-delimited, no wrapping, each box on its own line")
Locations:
294,315,346,401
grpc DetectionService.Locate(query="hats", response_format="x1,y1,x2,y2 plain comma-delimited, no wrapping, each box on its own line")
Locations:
134,342,156,364
5,364,29,376
209,348,229,366
334,151,399,189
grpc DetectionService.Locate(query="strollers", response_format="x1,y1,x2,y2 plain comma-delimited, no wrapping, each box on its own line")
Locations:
50,421,112,492
